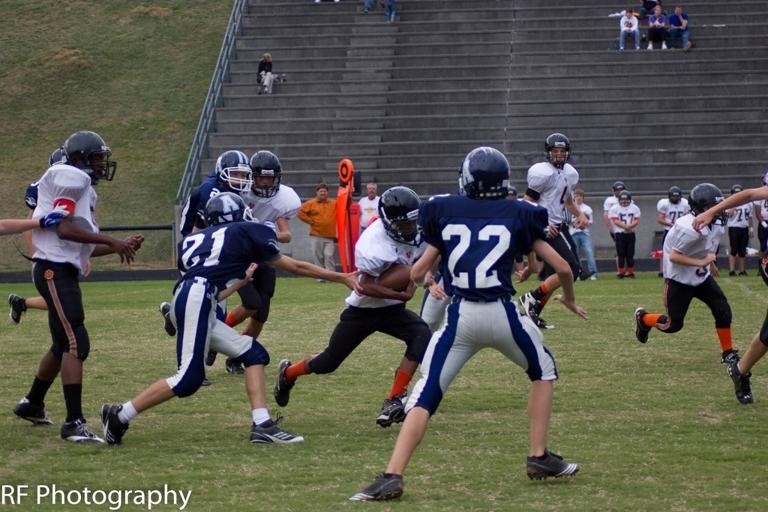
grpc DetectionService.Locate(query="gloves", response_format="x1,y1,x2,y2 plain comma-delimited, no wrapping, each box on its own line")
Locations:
40,202,70,227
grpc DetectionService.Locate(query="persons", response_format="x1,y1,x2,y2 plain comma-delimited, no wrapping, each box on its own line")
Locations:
361,0,376,15
0,201,71,237
639,1,663,20
646,5,670,50
380,0,397,24
11,129,146,447
154,149,768,427
345,142,579,502
100,187,365,451
618,7,641,51
3,146,70,327
255,53,275,95
518,130,592,328
667,5,697,51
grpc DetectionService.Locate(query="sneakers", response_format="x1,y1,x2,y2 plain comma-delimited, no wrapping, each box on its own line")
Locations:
226,359,245,373
376,393,408,427
721,348,753,404
274,359,295,406
348,472,403,501
159,302,176,336
580,271,595,280
519,290,554,329
635,307,651,343
14,396,129,444
527,448,579,480
8,293,22,323
250,412,304,443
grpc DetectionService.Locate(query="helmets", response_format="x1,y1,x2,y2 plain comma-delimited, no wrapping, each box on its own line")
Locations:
49,131,116,184
669,186,682,204
204,150,282,227
458,147,510,200
688,183,728,225
731,184,743,194
612,181,631,207
761,173,768,187
545,133,570,168
378,186,422,245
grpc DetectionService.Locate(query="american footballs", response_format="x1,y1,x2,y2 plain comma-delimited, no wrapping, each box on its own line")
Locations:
378,264,413,292
